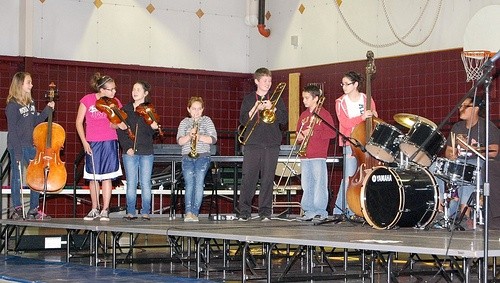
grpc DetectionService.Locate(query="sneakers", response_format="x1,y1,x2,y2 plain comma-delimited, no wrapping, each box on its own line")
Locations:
27,209,52,222
12,206,22,221
84,209,100,220
100,210,110,221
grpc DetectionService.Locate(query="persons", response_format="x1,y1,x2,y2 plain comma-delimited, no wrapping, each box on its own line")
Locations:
333,72,378,222
117,80,159,220
238,68,286,222
435,94,500,229
176,97,218,221
5,72,55,220
76,73,127,221
295,85,338,223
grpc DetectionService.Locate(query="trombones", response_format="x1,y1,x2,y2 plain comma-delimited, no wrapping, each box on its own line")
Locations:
274,95,325,194
237,80,287,147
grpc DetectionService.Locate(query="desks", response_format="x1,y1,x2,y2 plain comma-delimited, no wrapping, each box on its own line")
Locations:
138,154,338,220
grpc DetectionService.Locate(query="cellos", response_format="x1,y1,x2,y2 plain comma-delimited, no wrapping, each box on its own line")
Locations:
346,50,388,217
25,82,67,220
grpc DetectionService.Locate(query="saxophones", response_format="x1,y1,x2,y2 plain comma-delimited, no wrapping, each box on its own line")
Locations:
188,121,201,158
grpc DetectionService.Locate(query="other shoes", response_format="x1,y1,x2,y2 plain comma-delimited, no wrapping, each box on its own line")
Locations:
192,214,199,222
142,214,149,221
238,217,249,222
314,215,325,220
184,213,191,222
261,215,271,221
123,213,134,221
296,215,309,221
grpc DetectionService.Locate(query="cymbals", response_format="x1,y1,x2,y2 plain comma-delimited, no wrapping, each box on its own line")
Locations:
461,148,498,154
392,112,439,131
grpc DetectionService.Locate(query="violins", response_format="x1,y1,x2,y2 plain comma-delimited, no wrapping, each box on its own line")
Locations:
94,95,135,140
135,102,167,137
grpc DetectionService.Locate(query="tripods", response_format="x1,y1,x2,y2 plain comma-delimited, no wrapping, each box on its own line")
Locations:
424,183,465,231
312,113,366,226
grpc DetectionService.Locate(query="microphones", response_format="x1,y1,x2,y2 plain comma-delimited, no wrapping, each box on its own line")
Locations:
355,138,366,152
479,50,500,71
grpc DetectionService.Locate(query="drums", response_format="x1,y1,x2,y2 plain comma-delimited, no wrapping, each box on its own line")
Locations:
400,120,448,168
363,120,404,164
435,159,477,185
358,162,440,231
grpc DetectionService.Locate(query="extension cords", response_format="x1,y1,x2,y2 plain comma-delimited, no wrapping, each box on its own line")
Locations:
214,216,234,221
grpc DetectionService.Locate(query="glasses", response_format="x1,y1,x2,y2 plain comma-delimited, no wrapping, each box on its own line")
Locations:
102,86,117,91
340,82,354,86
